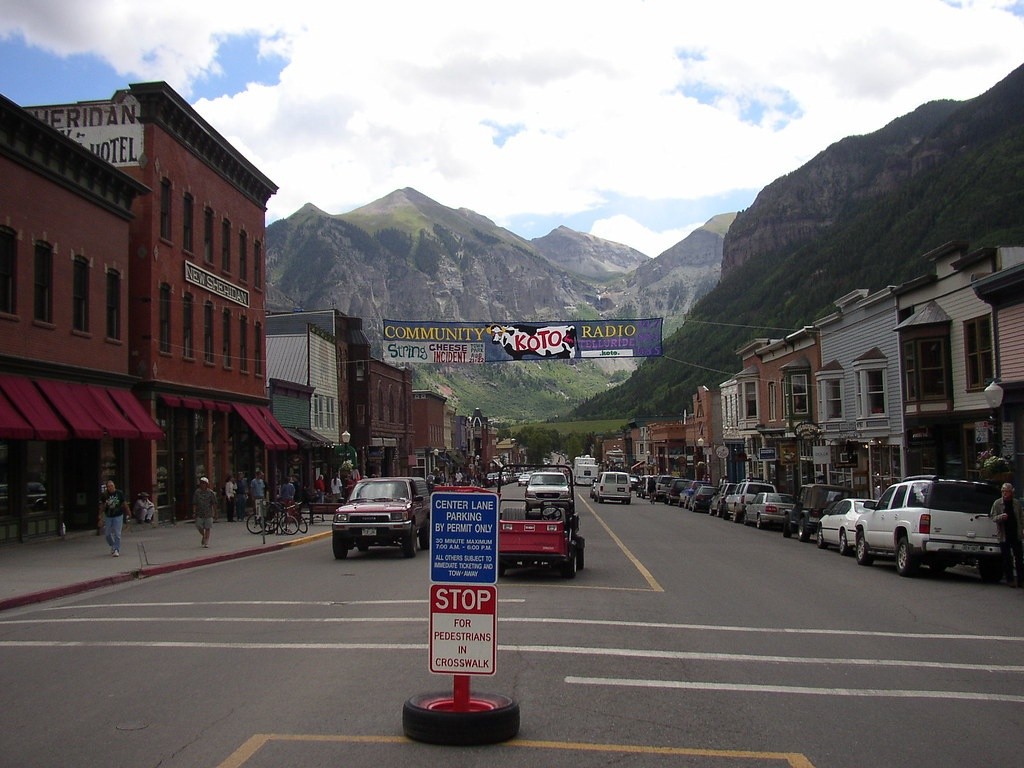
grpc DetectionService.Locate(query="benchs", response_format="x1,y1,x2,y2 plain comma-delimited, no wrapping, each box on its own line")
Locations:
308,501,343,523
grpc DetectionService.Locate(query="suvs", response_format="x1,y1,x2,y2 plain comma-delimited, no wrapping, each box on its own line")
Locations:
782,484,855,543
854,474,1008,578
332,476,430,560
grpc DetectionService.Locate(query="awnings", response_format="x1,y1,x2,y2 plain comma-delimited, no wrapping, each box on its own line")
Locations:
161,394,297,451
285,428,333,448
0,375,166,441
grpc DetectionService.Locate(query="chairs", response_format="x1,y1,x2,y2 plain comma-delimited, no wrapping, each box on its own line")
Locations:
502,507,525,520
543,508,566,523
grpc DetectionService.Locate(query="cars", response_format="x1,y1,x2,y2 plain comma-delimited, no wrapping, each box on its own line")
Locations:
485,465,541,487
629,471,778,524
743,491,798,530
817,497,889,555
0,480,50,514
523,470,574,512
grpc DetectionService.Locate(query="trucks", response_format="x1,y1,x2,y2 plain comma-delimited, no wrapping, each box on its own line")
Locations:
572,454,600,485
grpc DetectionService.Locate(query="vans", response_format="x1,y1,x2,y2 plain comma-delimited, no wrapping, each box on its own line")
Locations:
590,471,633,505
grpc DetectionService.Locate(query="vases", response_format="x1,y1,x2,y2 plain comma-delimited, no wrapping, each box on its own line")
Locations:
992,471,1012,483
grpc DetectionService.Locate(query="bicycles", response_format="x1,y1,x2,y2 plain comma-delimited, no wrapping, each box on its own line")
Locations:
246,500,309,535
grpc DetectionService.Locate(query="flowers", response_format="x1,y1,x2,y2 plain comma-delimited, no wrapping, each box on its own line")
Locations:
974,447,1014,478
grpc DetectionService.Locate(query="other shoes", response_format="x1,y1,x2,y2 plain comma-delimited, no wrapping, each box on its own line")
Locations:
204,544,208,548
138,519,144,524
111,544,119,557
145,518,151,523
1007,581,1016,588
201,536,204,545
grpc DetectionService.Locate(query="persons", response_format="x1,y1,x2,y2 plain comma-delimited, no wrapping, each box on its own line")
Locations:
235,471,248,521
292,474,300,502
331,474,342,496
250,471,265,524
225,475,238,522
192,477,218,548
315,475,325,493
352,467,360,482
991,483,1024,588
135,492,155,523
282,477,295,500
648,478,656,502
99,480,131,557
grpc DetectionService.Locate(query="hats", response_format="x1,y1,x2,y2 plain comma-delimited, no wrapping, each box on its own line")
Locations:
200,477,209,484
138,492,149,496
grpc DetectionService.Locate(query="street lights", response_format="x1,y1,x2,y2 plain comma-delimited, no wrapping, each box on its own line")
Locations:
434,448,439,468
984,380,1005,456
646,450,650,475
697,437,705,481
342,431,351,461
